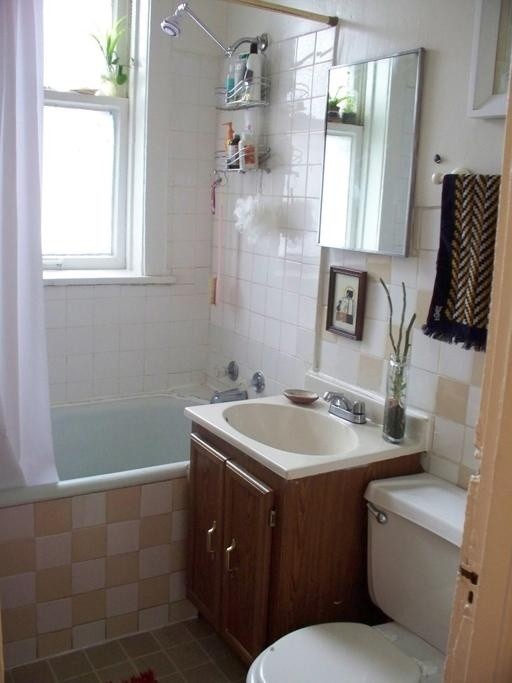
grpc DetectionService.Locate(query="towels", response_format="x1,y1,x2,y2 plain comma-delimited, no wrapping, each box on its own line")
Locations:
421,172,501,351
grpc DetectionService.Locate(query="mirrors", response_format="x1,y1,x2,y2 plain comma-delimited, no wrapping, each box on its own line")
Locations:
316,45,427,259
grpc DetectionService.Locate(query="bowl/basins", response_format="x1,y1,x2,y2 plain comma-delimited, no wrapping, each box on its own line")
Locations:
284,388,319,403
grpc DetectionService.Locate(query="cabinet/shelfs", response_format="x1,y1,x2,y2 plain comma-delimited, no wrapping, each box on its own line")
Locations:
182,430,428,671
210,71,273,177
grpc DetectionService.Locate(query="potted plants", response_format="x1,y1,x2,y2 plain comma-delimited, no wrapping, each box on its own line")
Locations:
90,13,135,96
326,84,350,122
375,275,420,447
341,95,358,124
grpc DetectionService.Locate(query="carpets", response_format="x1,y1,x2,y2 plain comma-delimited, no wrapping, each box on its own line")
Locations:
106,665,159,682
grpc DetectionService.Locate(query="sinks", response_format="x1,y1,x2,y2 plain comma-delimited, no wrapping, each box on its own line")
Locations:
184,389,428,480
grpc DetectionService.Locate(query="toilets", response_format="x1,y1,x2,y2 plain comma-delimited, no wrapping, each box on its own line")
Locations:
244,470,468,683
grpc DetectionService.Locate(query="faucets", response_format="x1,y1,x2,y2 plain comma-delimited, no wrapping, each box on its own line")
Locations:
211,386,250,405
322,391,353,411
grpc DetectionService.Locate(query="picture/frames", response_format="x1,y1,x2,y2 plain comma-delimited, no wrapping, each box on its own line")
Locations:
323,264,367,343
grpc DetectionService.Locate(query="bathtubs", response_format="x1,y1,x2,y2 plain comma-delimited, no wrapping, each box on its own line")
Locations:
0,389,212,510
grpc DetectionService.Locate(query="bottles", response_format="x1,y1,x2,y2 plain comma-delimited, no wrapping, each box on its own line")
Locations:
228,131,242,169
